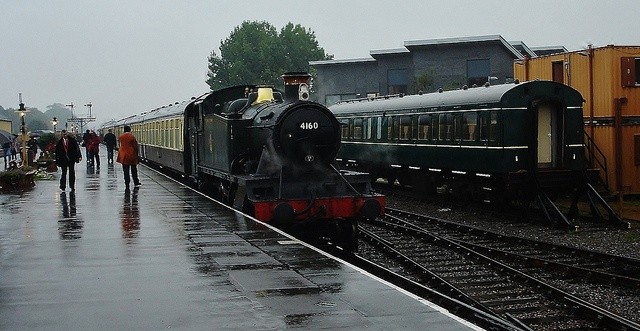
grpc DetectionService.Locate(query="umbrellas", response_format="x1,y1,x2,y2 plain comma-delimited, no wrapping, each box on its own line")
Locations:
0,129,42,145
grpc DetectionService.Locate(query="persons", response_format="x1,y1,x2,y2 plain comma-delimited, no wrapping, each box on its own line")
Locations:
83,130,92,147
55,131,82,192
3,138,38,163
104,130,116,164
87,130,101,167
117,126,140,186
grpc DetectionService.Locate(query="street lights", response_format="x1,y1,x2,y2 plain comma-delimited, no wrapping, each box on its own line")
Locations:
14,102,30,170
50,116,59,134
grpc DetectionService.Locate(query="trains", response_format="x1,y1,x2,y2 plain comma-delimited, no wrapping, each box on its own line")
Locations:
328,79,632,232
97,72,385,222
